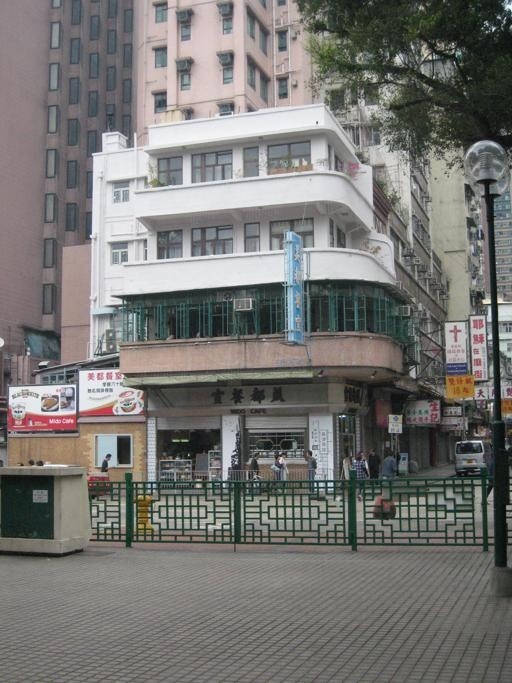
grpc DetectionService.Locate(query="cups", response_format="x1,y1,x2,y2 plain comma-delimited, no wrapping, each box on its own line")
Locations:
116,387,143,411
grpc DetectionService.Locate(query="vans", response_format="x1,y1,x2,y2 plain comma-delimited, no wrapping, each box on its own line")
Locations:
454,440,495,478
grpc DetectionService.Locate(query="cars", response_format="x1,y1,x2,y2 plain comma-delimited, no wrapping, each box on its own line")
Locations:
87,472,112,498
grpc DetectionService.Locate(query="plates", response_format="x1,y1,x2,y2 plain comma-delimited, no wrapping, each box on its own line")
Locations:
41,398,58,411
112,401,144,416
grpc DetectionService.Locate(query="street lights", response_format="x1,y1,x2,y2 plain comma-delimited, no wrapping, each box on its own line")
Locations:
461,139,510,570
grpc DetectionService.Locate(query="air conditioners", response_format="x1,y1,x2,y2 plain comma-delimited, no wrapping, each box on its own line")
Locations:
177,9,191,24
175,61,191,72
217,2,231,15
104,343,120,353
105,327,123,340
398,304,414,317
233,298,256,312
218,52,233,67
219,105,233,115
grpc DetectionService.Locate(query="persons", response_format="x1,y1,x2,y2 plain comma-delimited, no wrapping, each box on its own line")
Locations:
271,453,284,493
305,450,317,494
335,447,401,502
101,453,116,471
479,441,493,505
248,453,260,479
407,455,419,478
0,459,53,467
279,452,290,493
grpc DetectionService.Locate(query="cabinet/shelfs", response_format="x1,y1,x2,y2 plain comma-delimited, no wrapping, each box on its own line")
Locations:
158,458,193,489
208,450,223,488
